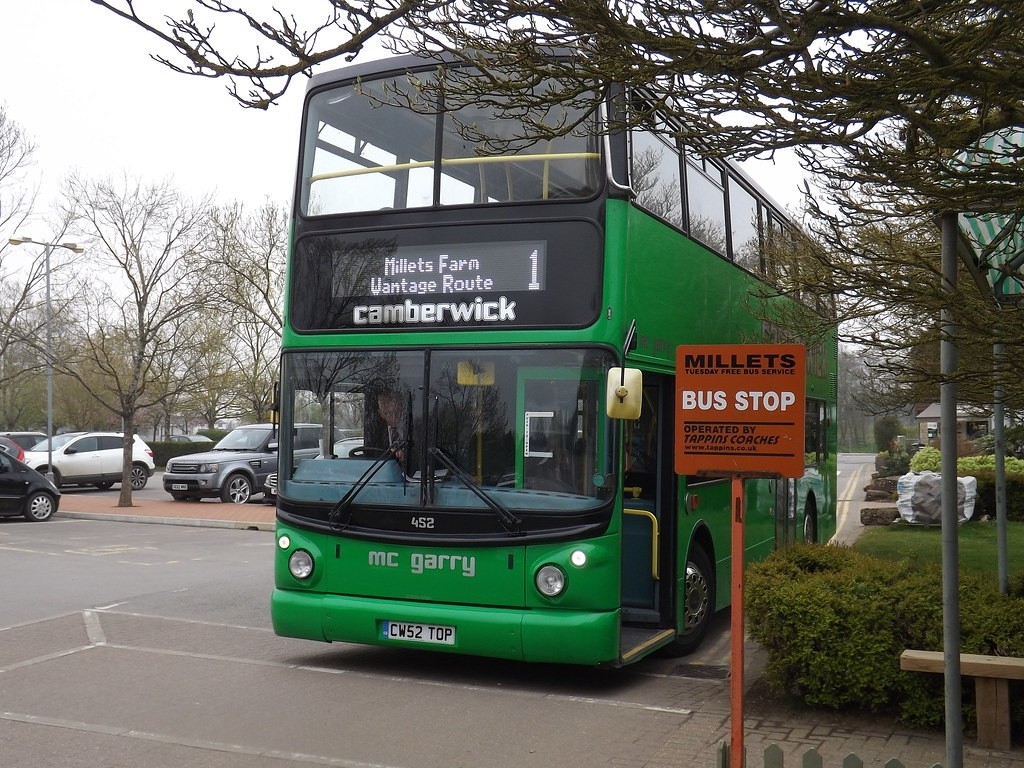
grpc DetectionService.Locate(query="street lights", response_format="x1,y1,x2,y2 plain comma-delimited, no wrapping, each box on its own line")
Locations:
8,236,84,486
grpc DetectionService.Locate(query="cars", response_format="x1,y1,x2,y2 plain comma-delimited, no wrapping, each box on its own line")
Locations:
264,437,364,507
163,423,348,504
0,450,62,522
0,436,25,462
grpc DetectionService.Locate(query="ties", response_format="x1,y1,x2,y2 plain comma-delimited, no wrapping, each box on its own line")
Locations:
390,428,400,445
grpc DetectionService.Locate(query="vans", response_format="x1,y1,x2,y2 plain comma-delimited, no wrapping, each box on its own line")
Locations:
0,432,47,449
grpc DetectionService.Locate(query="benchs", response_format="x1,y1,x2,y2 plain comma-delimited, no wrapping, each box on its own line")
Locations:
899,649,1024,750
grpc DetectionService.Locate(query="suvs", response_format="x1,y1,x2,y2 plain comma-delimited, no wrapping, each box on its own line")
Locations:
22,429,156,490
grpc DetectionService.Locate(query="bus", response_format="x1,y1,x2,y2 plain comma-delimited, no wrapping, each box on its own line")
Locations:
270,42,837,666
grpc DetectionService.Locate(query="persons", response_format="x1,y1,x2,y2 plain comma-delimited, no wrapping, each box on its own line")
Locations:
376,392,416,475
